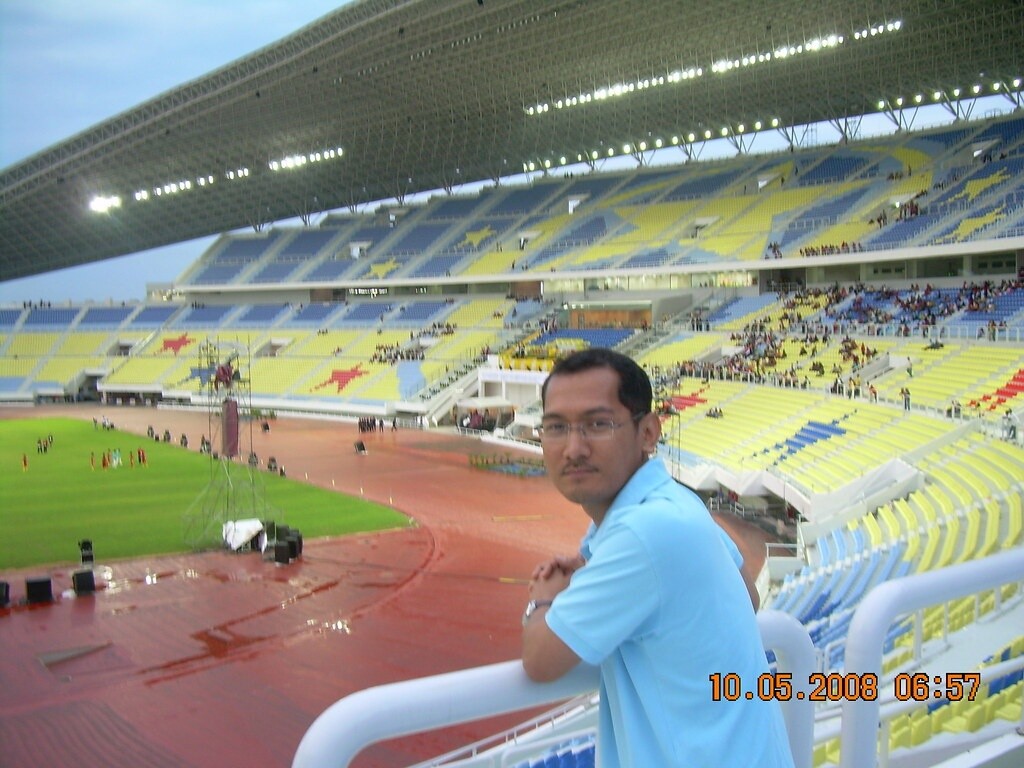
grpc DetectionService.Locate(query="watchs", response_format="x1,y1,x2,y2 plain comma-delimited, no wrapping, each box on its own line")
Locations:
522,599,553,629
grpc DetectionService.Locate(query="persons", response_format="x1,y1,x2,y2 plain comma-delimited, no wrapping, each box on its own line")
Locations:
12,154,1024,474
522,347,798,768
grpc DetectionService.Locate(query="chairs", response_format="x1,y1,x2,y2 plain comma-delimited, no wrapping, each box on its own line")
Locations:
0,112,1024,768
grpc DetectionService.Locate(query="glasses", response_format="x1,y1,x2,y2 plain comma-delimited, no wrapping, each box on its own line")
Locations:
532,412,646,444
